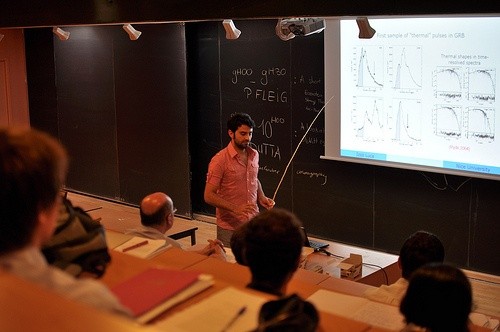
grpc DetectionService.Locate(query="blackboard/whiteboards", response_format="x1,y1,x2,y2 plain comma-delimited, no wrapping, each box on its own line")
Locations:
185,17,500,283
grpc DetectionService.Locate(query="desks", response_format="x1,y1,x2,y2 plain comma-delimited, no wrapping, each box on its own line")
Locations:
0,227,500,332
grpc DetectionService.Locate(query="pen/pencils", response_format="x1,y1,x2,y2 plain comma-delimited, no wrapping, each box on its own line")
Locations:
208,242,222,256
220,306,247,332
123,238,148,252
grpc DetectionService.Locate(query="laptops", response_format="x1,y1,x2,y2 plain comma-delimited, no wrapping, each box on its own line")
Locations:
300,227,329,251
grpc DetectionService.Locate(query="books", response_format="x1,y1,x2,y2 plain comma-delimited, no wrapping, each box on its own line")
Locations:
114,236,172,261
300,244,316,270
112,266,218,327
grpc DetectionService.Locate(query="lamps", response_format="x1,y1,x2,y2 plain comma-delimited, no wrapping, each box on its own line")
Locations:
51,17,376,40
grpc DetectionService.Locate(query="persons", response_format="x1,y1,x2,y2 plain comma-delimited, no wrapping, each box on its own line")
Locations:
242,210,304,295
369,231,445,307
399,265,491,332
125,192,226,259
0,125,131,316
204,114,275,249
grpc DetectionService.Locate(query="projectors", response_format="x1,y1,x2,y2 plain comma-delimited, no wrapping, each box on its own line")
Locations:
280,18,325,36
